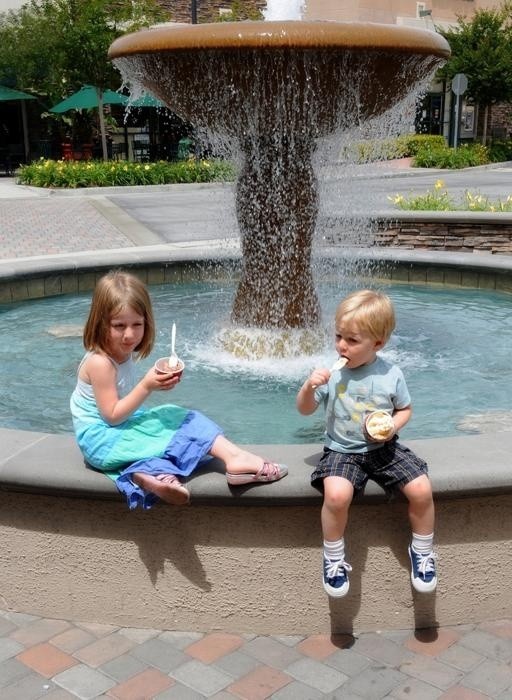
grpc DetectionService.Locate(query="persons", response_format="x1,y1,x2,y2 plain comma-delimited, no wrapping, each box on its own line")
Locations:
300,289,438,599
71,267,297,508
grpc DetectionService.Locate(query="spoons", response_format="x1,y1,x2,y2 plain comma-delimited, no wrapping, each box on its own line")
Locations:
169,323,180,369
312,356,348,390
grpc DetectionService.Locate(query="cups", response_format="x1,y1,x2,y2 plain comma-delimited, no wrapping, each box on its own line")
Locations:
155,357,185,384
363,410,395,442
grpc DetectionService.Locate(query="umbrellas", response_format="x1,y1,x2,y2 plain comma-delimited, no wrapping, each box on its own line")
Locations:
0,83,198,159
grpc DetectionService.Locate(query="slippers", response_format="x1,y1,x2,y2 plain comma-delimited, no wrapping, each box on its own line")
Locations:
134,472,191,508
226,460,289,485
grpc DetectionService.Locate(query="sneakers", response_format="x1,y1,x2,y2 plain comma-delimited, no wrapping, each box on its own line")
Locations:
408,545,439,596
322,552,353,599
